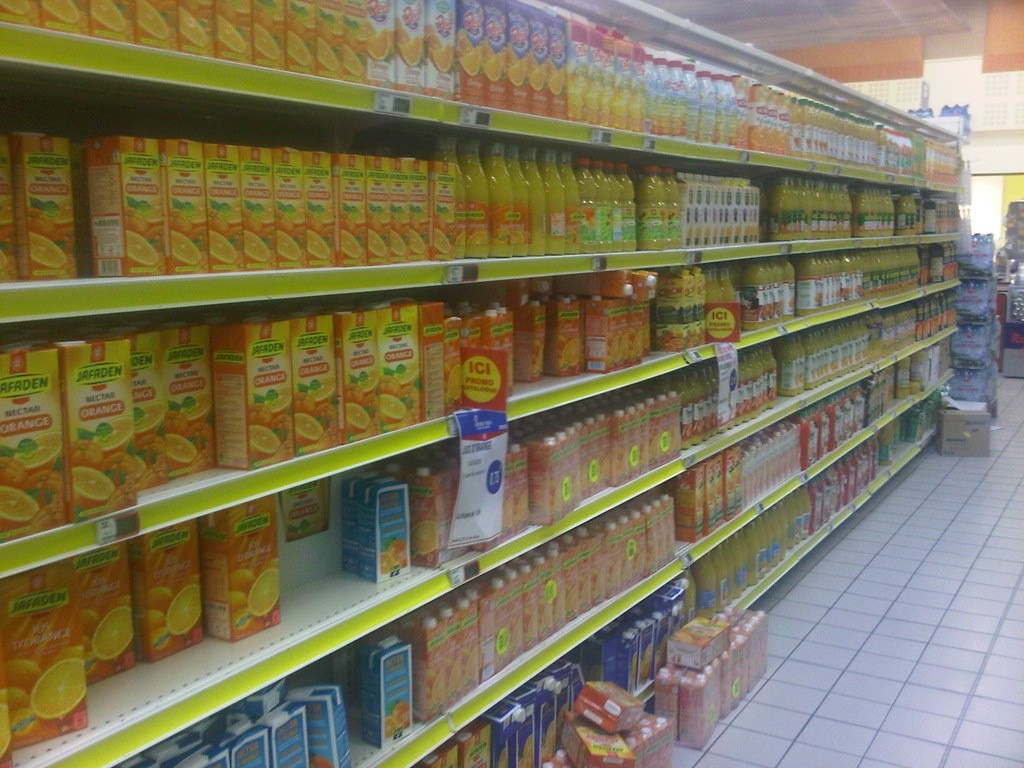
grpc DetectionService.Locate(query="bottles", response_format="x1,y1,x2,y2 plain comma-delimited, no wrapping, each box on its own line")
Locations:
416,20,998,722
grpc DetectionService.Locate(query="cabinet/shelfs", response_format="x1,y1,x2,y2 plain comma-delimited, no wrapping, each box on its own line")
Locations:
2,20,967,767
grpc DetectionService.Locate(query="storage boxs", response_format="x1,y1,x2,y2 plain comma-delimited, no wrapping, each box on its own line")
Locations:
936,409,990,458
